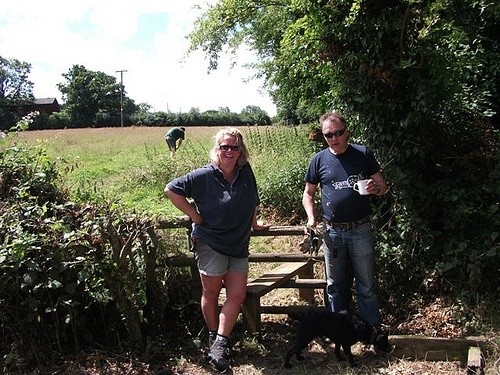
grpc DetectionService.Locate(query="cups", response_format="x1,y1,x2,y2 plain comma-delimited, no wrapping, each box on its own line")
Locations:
353,180,370,195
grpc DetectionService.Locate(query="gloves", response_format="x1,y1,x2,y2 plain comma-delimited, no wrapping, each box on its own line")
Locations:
298,234,313,254
305,224,325,257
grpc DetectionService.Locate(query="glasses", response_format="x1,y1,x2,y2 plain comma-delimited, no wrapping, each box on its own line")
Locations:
323,127,346,138
218,145,240,151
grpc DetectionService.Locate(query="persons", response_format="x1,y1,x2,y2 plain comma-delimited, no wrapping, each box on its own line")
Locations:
302,111,391,352
164,127,185,152
163,128,273,374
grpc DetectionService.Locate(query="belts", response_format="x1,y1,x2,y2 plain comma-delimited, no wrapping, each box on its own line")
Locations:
322,216,370,228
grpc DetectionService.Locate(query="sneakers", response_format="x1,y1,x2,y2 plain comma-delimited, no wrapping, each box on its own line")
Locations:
208,335,231,357
205,341,232,374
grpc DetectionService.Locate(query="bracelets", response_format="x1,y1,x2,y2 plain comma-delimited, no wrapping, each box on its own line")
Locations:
375,184,381,194
